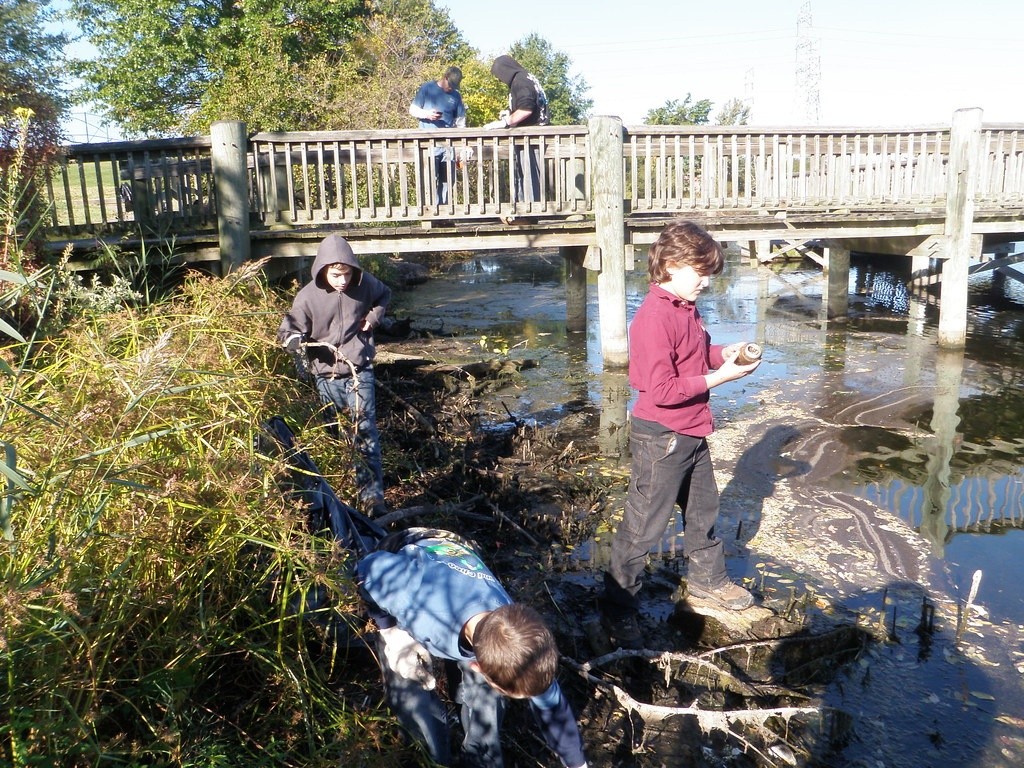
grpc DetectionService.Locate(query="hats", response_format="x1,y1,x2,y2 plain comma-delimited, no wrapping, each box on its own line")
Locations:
445,66,463,91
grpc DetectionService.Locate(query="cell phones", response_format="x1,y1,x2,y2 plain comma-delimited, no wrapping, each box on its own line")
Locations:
437,112,443,115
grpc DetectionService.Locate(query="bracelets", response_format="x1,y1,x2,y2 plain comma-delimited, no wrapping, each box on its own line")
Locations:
503,117,509,126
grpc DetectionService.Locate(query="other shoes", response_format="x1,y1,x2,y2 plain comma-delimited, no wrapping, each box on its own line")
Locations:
432,220,456,228
510,216,538,225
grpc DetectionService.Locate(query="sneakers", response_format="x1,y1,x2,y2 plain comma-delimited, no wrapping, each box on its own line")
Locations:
359,497,388,519
604,613,645,651
686,579,754,612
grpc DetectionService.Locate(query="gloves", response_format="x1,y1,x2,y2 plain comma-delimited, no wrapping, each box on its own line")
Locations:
377,627,438,692
483,110,510,131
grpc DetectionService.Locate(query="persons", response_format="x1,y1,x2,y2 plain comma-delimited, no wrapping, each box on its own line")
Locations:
276,234,394,519
353,527,591,768
598,220,762,649
483,53,550,207
407,67,469,205
